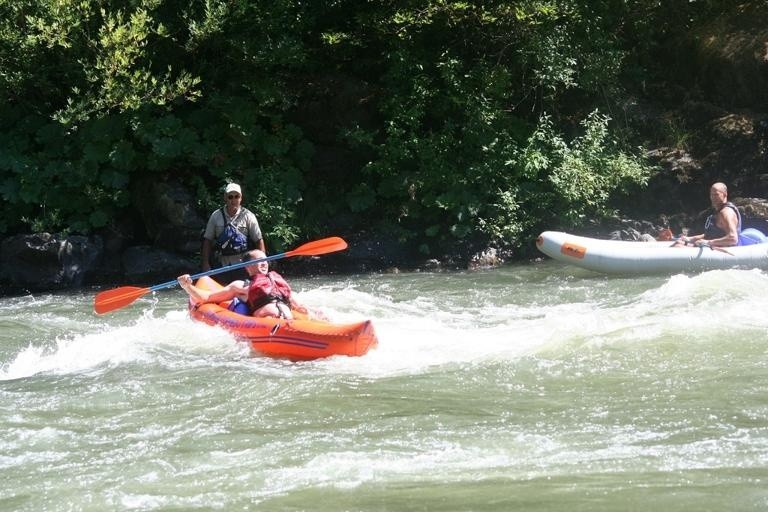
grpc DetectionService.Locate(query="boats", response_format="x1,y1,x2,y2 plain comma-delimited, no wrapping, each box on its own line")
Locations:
535,229,767,275
188,274,378,360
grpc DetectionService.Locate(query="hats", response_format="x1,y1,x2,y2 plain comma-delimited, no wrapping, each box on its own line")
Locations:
224,183,242,195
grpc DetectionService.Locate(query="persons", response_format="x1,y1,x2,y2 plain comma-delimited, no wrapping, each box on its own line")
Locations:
201,181,266,283
177,248,296,321
680,182,742,248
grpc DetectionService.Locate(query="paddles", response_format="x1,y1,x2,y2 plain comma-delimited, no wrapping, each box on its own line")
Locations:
660,228,736,258
95,235,347,314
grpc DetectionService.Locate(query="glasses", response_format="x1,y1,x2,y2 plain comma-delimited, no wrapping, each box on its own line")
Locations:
228,195,240,199
